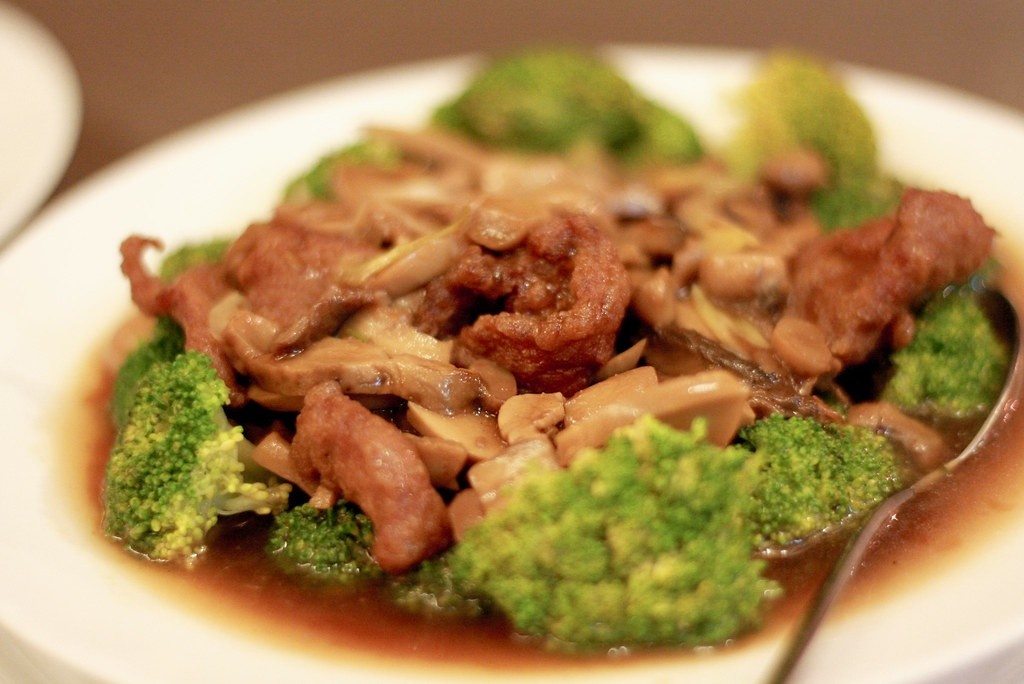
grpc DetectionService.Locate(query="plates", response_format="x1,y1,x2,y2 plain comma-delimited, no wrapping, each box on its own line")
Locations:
0,0,1024,684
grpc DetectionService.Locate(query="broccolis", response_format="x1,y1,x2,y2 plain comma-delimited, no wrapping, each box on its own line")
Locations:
95,48,1014,647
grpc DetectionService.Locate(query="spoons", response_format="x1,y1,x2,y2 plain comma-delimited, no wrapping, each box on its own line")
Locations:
762,282,1020,684
